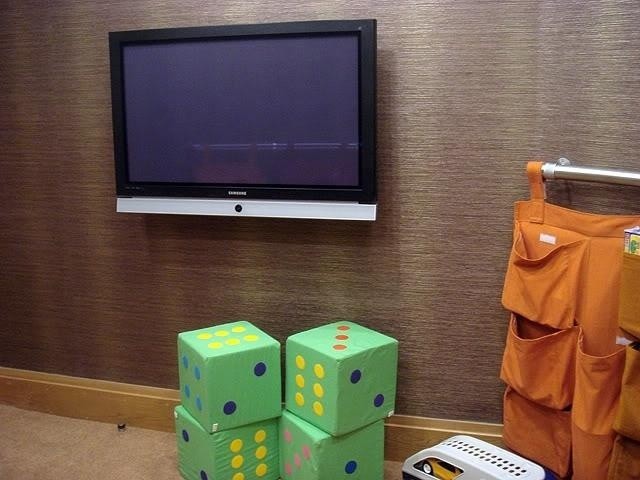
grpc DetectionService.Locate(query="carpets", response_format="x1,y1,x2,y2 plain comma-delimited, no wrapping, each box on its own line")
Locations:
0,400,403,480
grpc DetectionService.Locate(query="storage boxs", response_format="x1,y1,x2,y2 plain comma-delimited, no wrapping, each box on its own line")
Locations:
401,435,558,480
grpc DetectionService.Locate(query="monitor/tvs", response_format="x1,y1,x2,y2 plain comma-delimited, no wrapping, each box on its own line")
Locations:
108,19,379,222
